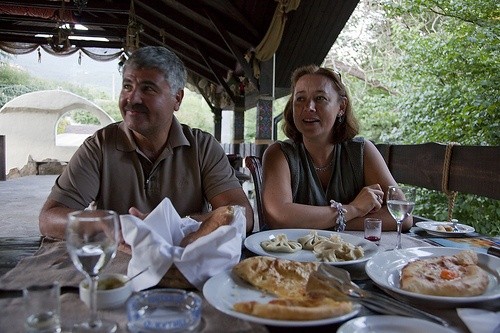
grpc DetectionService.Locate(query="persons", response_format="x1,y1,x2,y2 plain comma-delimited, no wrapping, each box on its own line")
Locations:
36,46,255,244
259,64,413,233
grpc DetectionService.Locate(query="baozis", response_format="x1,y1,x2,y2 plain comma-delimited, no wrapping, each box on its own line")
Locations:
260,230,365,263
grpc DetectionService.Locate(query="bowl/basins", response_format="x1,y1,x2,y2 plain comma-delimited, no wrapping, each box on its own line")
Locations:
79,274,132,310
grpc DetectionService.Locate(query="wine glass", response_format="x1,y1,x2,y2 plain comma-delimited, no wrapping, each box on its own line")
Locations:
386,185,416,252
66,208,120,333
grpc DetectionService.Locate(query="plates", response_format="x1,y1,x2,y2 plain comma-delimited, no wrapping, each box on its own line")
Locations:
365,246,500,308
244,229,380,265
337,313,458,333
415,221,475,238
203,269,364,326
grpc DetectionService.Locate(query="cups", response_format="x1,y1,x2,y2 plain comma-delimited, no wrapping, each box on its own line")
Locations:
24,279,62,333
364,218,382,246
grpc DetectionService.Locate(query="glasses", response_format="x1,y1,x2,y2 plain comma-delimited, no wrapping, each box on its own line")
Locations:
323,67,341,82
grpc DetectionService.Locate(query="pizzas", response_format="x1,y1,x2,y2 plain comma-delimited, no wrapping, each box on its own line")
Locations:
231,255,352,319
400,254,489,297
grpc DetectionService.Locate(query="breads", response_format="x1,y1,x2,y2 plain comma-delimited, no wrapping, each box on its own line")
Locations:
179,206,234,247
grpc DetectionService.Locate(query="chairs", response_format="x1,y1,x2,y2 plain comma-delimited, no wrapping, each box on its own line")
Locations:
245,156,270,233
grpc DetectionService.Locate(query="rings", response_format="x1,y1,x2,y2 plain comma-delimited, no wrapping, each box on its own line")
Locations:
376,194,381,200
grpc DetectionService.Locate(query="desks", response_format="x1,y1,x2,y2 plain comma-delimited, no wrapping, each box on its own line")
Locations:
0,229,500,333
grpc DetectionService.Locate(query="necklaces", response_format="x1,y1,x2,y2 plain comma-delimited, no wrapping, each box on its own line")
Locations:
315,161,331,172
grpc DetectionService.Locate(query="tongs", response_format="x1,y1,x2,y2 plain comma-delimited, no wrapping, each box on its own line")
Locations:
305,262,449,327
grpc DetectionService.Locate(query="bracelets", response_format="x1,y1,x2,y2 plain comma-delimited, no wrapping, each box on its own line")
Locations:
329,199,348,232
